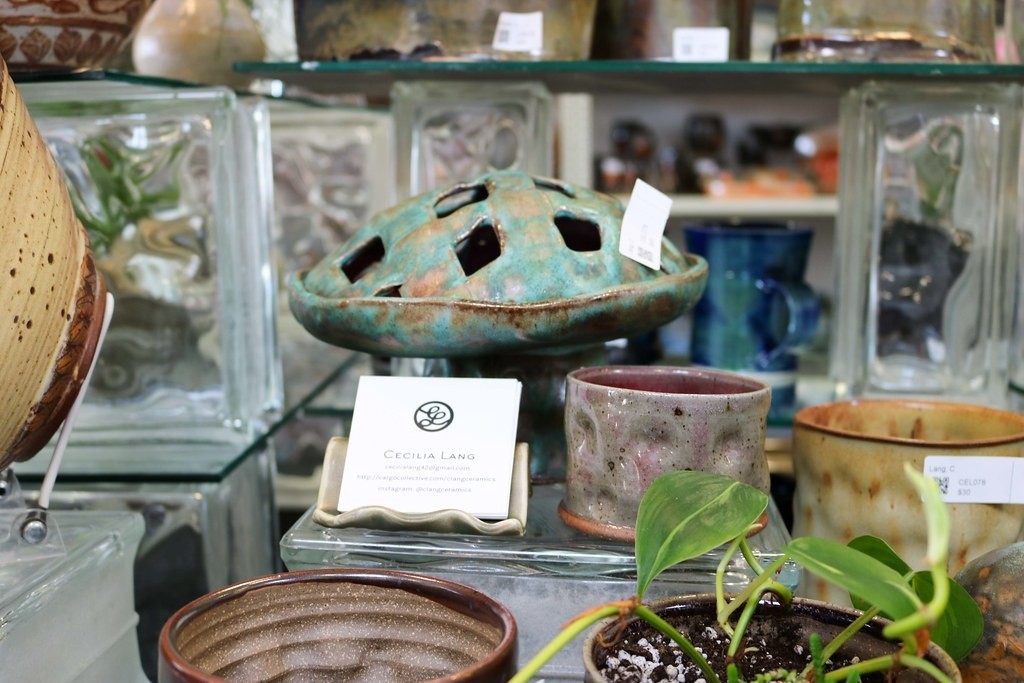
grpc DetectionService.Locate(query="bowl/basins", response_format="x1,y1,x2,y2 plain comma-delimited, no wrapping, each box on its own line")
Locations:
2,53,108,473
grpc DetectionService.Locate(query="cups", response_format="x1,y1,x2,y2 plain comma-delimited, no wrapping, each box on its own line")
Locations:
685,216,826,408
